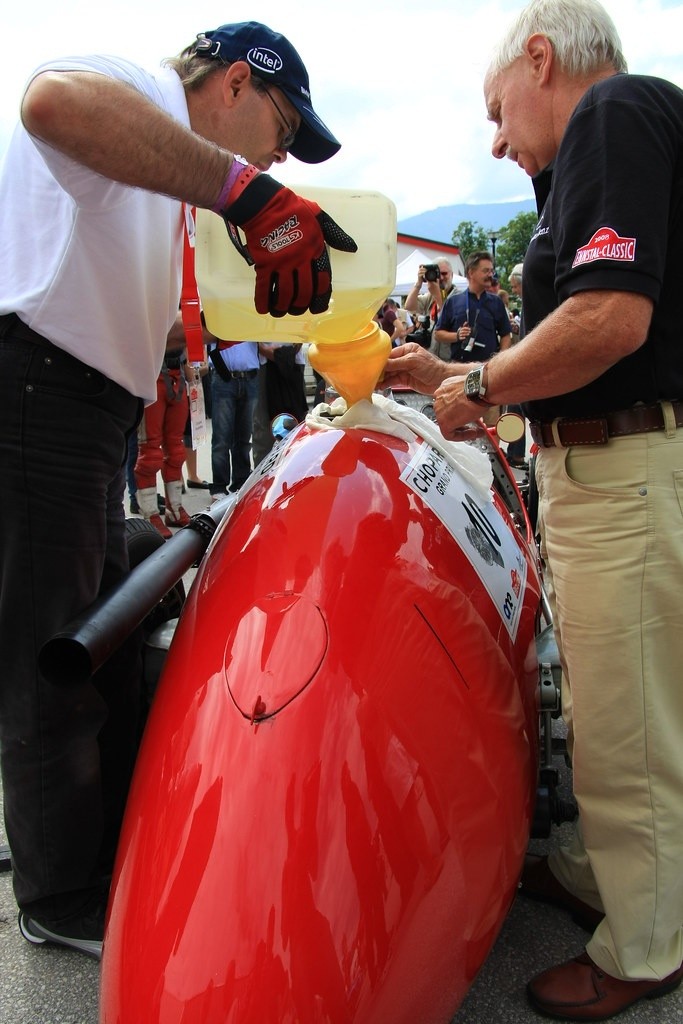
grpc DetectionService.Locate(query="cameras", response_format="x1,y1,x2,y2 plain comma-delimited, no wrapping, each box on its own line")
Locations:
419,264,440,282
405,315,432,348
461,327,486,351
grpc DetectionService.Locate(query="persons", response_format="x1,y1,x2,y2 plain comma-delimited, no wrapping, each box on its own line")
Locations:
1,20,342,960
122,251,525,539
375,0,683,1024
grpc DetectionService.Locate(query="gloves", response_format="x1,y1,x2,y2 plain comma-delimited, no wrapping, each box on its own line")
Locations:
224,171,358,318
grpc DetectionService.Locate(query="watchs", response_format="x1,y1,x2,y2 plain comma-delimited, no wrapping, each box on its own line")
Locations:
464,363,497,409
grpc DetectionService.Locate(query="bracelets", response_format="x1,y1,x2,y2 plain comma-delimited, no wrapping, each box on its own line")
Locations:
213,154,261,214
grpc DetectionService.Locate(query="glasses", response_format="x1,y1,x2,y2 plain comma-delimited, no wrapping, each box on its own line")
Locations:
472,267,497,276
250,70,296,150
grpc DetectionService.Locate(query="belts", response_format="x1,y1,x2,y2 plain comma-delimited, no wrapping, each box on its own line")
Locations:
529,403,683,449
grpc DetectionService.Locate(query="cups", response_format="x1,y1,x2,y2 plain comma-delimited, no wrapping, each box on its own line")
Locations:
325,386,340,406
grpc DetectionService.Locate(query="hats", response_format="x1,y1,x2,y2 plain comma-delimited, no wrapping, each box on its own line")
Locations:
197,21,342,164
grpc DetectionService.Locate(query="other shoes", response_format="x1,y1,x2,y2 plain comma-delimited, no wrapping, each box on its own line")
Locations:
517,856,606,934
19,902,107,958
158,494,166,505
212,493,224,501
130,500,167,515
525,943,683,1022
187,478,212,489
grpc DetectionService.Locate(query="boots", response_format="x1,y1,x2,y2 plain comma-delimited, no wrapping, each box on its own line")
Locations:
135,488,172,538
164,480,194,527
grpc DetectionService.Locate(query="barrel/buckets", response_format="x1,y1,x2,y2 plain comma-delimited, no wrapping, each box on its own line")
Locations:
194,187,396,342
